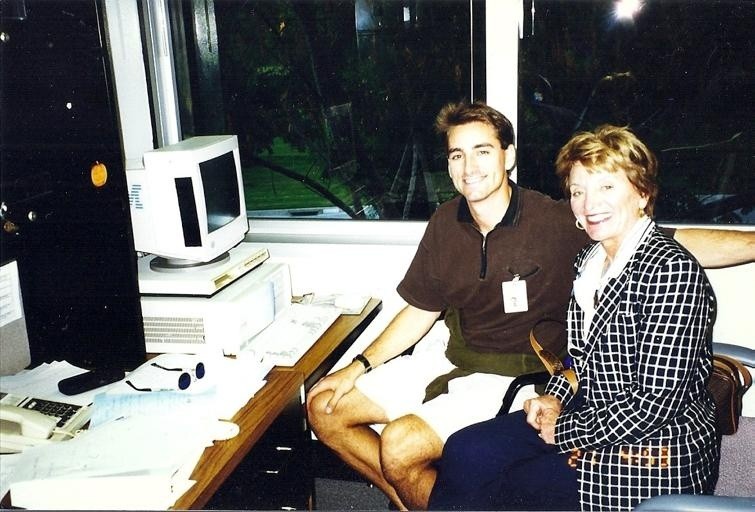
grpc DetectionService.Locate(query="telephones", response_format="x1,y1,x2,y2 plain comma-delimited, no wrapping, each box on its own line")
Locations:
0,391,96,454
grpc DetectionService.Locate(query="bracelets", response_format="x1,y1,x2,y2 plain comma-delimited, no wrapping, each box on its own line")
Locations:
353,353,372,374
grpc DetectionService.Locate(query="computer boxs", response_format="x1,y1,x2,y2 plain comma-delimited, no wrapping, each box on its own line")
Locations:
140,261,293,358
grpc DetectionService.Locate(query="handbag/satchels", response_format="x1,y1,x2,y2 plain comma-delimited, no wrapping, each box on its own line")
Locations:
707,355,751,435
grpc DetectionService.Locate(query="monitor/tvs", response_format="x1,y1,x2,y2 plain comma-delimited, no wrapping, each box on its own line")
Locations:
124,132,251,272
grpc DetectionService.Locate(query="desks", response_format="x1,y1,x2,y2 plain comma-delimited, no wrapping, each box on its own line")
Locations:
0,295,383,511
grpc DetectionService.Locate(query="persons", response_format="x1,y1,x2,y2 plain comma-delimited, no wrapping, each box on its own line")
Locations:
305,102,755,510
420,125,719,510
